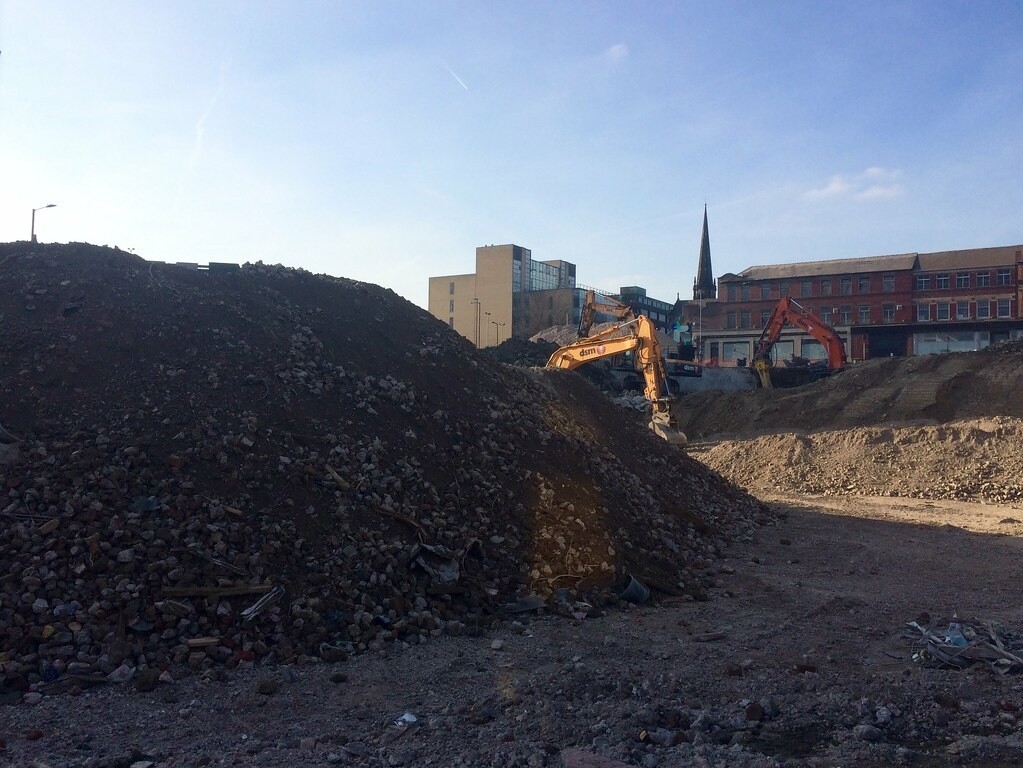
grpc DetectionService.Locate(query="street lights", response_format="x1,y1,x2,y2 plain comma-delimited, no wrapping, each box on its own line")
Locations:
485,311,491,346
498,323,506,342
473,297,477,346
30,203,57,242
468,301,481,348
492,321,499,346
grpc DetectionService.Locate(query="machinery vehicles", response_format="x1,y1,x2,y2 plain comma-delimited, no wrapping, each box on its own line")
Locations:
751,295,852,388
542,312,689,456
576,289,703,396
